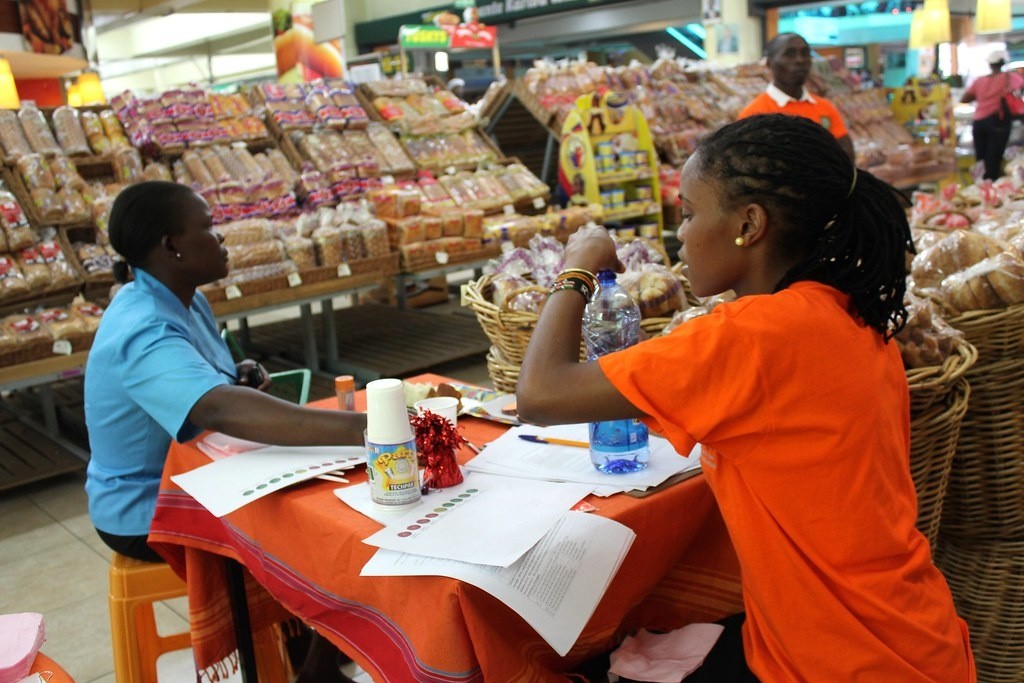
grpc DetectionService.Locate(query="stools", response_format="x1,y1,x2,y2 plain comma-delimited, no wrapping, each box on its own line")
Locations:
107,546,296,683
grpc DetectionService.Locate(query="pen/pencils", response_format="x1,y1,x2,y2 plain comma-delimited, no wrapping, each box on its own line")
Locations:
519,435,588,448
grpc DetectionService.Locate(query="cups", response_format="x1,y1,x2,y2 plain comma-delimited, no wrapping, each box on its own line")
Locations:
363,426,422,506
414,396,460,428
366,378,412,440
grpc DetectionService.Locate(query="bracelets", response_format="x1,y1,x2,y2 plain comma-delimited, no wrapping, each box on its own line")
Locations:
549,268,601,303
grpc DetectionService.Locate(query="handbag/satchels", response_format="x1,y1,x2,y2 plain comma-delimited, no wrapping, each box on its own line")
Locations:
1001,72,1024,120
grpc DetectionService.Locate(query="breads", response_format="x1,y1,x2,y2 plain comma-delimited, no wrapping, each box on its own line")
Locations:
0,74,604,370
487,152,1024,369
524,57,933,204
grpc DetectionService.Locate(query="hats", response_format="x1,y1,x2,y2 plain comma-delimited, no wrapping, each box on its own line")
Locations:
989,52,1006,64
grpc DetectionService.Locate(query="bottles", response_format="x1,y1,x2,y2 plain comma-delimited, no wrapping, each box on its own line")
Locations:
579,266,651,475
334,375,355,412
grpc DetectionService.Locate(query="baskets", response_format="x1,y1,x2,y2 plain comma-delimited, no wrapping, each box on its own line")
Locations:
460,259,1023,683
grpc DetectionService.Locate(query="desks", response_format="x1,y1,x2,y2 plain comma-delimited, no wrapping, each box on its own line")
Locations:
145,372,745,683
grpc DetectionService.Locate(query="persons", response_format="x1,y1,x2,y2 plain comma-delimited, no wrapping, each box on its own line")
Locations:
960,52,1024,180
739,32,854,165
516,112,977,683
83,181,416,683
